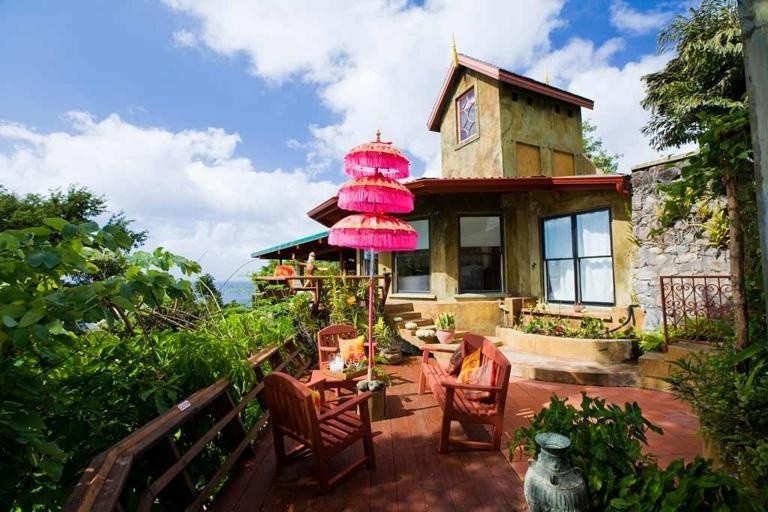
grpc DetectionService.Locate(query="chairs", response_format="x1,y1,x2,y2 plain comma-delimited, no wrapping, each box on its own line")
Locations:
262,371,378,494
317,323,379,396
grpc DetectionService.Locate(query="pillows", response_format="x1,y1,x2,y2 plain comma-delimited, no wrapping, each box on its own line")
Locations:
336,333,366,364
301,382,321,419
445,341,495,402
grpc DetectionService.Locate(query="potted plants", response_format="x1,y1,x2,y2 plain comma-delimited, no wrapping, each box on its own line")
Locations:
436,311,456,344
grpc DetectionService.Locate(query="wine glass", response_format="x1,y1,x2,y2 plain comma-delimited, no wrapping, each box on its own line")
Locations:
344,357,359,369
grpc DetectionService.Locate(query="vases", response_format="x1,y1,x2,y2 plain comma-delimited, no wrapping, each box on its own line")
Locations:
522,431,588,512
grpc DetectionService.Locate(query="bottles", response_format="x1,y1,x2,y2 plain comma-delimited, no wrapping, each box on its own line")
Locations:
335,349,341,361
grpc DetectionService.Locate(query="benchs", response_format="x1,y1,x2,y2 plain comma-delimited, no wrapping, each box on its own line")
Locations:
416,334,511,454
